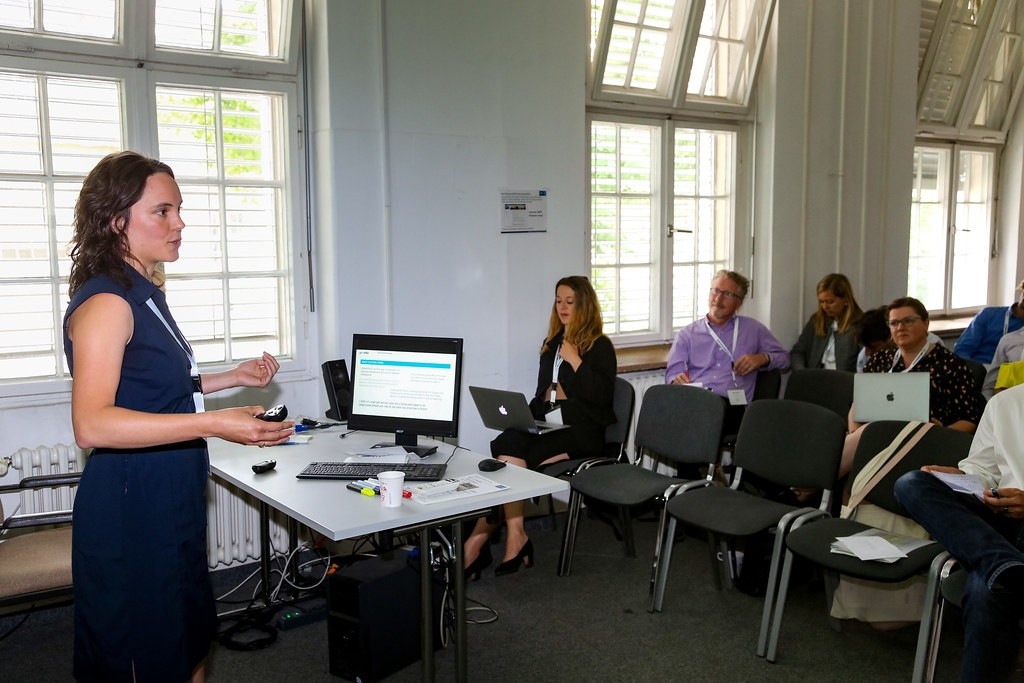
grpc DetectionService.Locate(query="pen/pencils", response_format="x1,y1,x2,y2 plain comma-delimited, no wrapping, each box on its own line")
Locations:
684,361,689,377
991,488,999,498
277,442,299,445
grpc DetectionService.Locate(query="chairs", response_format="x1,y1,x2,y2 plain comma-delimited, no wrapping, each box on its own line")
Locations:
763,422,974,683
538,375,634,574
720,371,781,449
0,472,86,623
926,557,975,681
654,399,844,656
568,383,729,596
784,367,858,415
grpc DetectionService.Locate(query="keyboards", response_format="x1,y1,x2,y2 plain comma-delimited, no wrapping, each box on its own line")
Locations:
295,461,447,481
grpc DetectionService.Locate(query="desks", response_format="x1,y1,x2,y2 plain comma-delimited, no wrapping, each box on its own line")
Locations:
206,416,571,683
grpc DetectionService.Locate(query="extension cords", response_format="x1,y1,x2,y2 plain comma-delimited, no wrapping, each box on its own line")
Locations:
276,606,327,630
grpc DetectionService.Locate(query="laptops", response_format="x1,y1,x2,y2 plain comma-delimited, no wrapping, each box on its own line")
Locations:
469,385,571,434
853,373,930,424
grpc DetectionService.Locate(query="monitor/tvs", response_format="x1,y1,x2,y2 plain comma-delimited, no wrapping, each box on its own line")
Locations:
347,333,464,457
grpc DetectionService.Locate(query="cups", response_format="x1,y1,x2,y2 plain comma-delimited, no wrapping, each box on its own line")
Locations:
376,471,405,507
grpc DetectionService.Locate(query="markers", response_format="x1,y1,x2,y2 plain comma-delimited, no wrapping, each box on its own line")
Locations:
347,480,380,496
369,478,412,498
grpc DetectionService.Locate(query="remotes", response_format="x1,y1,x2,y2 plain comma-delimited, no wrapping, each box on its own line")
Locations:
252,460,277,474
256,404,288,422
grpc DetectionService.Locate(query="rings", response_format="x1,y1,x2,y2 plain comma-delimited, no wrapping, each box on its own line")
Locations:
1004,506,1008,514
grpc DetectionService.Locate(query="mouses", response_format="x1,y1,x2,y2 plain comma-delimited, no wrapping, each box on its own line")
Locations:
478,459,506,472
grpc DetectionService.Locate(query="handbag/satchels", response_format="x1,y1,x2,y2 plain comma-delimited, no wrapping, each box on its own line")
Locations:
830,419,937,621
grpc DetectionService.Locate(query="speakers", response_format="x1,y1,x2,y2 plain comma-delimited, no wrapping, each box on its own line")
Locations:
322,359,350,421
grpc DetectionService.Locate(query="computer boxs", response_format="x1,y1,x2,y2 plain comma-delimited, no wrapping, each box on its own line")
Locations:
323,544,447,683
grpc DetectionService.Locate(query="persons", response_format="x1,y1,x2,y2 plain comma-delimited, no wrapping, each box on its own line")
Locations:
894,380,1024,683
665,271,791,541
463,275,618,582
791,274,864,371
847,306,945,374
63,152,296,683
952,280,1024,402
789,297,987,505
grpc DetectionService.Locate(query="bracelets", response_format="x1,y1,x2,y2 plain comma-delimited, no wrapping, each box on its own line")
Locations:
765,353,771,362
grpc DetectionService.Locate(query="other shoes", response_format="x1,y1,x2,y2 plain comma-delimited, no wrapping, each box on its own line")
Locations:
769,488,801,507
698,464,729,487
663,526,687,541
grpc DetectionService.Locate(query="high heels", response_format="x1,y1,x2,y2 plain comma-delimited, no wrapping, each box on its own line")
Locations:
463,554,482,582
495,538,534,575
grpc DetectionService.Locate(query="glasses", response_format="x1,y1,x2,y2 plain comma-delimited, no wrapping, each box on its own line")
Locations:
886,316,925,327
710,288,741,299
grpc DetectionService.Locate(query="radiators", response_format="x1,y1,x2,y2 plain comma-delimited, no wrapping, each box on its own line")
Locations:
10,439,322,570
